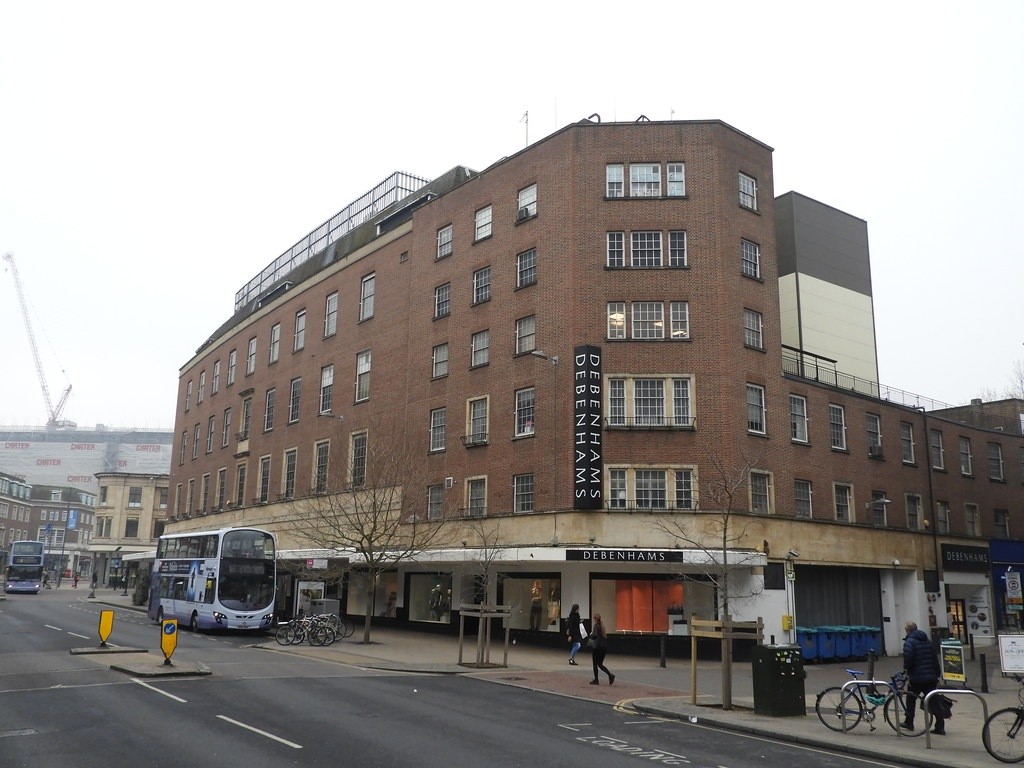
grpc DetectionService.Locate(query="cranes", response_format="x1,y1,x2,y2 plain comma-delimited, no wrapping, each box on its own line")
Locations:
3,250,78,429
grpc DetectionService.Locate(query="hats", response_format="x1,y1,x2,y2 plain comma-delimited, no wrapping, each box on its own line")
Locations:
432,589,435,591
436,585,440,587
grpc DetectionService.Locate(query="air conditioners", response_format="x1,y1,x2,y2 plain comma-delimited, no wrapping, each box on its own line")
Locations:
871,446,882,456
517,208,528,219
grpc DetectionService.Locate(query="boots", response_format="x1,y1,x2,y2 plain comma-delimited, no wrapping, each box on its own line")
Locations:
550,620,556,625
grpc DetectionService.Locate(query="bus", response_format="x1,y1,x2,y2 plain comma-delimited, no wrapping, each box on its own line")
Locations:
3,540,47,593
148,526,278,632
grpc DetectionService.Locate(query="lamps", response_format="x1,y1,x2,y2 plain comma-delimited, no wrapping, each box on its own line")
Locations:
787,550,798,560
531,351,559,366
866,499,892,509
894,558,900,565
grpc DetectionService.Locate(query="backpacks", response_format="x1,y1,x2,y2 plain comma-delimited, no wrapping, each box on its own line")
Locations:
920,681,957,719
565,617,570,635
76,575,80,581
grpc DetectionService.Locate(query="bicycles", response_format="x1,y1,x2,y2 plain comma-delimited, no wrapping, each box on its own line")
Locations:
276,613,355,647
816,668,933,737
982,673,1024,764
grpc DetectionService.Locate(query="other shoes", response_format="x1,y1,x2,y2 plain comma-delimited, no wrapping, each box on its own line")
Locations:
533,627,539,631
528,627,534,630
899,722,914,730
609,674,615,685
568,658,573,663
930,728,945,735
569,661,578,665
589,680,599,685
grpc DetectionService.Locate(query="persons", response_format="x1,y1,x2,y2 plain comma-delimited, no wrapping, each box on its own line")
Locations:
42,571,49,588
548,582,560,626
120,571,127,589
900,621,947,736
588,612,615,685
429,584,451,621
530,581,543,631
93,569,99,589
565,603,588,664
72,573,79,589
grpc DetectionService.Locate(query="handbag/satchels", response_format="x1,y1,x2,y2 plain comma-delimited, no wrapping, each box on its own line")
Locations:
579,620,587,639
586,633,599,650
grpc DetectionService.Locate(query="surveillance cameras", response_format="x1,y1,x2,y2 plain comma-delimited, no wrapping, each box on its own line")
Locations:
894,560,900,566
789,551,800,557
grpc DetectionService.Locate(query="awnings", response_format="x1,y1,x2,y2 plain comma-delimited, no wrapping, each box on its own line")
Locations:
122,550,157,560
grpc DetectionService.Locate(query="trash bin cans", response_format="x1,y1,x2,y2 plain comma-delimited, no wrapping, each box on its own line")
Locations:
797,624,882,665
749,643,807,716
